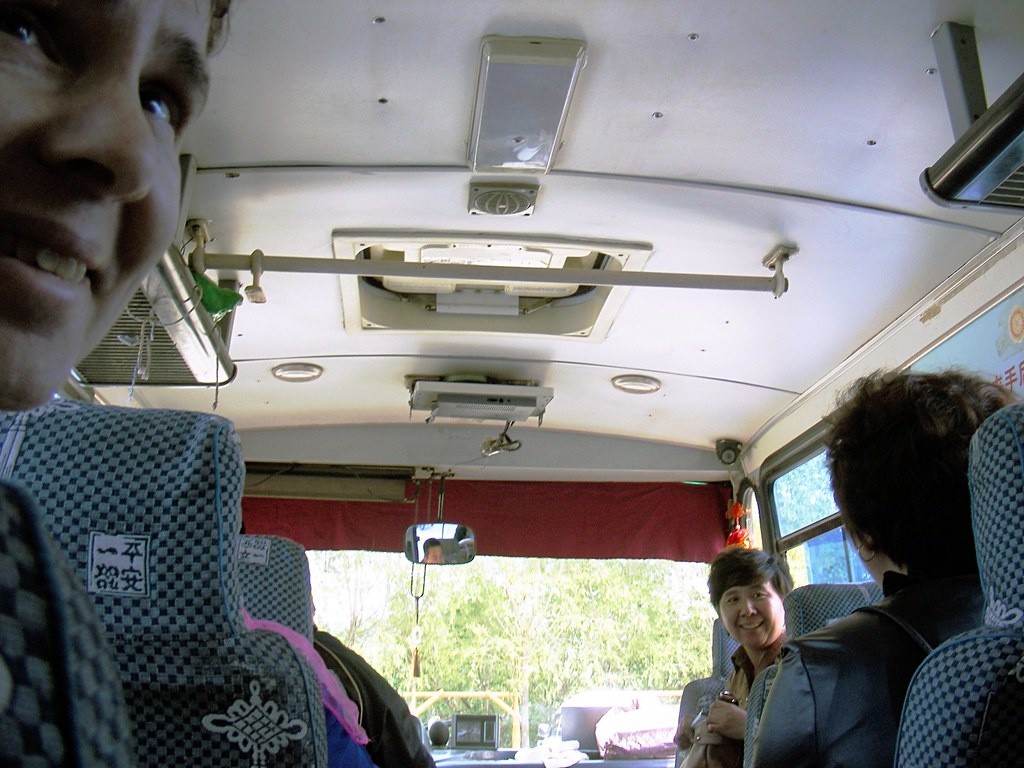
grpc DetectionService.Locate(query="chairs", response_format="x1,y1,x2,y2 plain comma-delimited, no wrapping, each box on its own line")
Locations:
0,400,329,768
742,581,885,768
675,617,742,768
893,402,1024,768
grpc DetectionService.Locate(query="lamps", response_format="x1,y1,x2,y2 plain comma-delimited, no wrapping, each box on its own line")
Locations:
467,34,588,175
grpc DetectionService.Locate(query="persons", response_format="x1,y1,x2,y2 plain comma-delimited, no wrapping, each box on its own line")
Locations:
454,526,474,557
0,0,234,768
422,538,445,564
671,547,795,768
311,595,437,768
741,366,1016,768
239,609,377,768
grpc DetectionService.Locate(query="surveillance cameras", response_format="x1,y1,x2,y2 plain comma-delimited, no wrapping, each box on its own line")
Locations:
715,439,742,464
